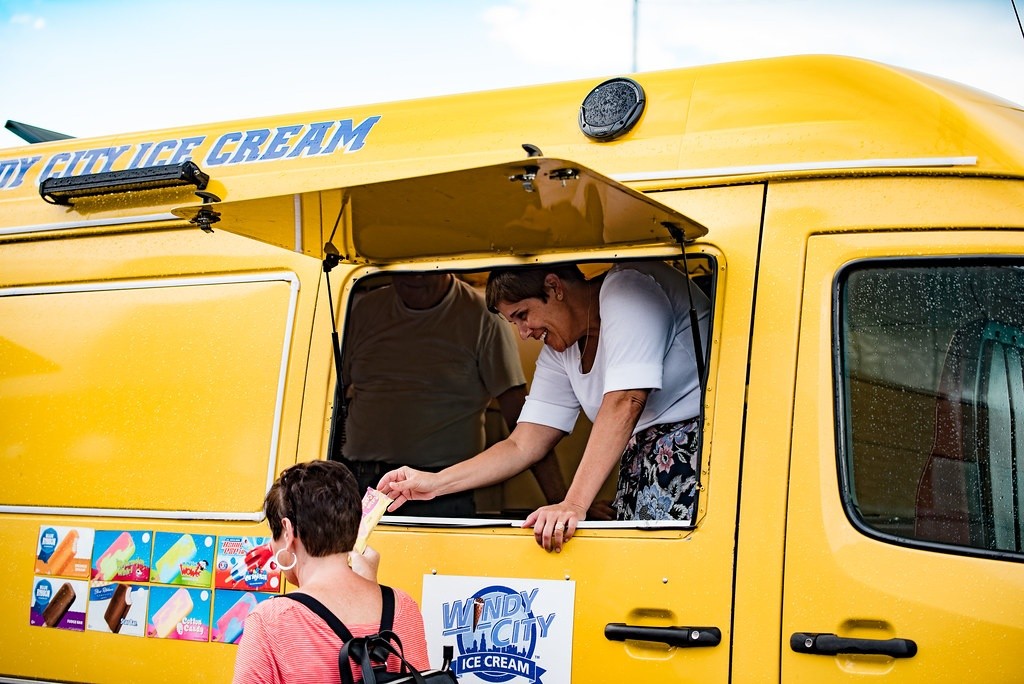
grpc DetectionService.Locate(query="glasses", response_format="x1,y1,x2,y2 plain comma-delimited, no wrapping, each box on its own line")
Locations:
280,464,301,538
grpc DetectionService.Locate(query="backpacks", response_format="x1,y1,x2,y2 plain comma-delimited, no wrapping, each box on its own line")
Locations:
274,584,459,684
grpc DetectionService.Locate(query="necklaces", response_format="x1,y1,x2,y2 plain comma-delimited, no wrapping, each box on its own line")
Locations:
575,285,593,359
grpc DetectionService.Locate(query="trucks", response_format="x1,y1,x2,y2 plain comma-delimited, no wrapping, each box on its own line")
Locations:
0,54,1024,684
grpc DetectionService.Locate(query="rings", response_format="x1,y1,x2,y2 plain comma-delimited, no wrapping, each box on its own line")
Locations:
555,524,565,531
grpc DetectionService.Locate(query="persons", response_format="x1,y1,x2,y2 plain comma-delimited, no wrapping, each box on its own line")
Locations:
376,261,711,555
233,460,431,684
339,269,615,522
